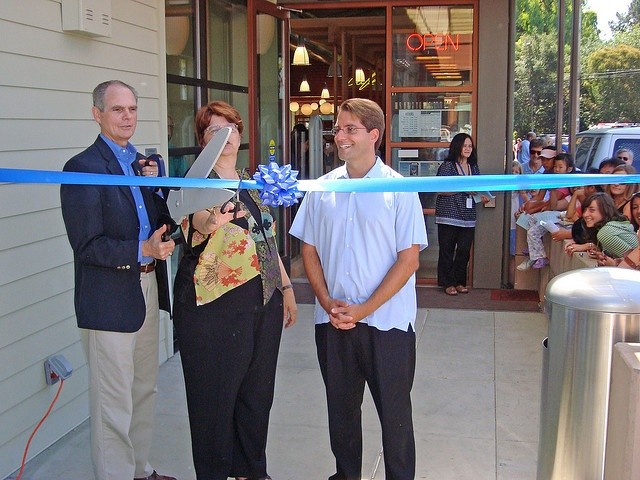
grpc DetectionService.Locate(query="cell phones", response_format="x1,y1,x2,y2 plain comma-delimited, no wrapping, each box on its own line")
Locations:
590,252,596,256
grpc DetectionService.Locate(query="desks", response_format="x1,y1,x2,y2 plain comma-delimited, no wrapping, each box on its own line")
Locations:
515,223,599,309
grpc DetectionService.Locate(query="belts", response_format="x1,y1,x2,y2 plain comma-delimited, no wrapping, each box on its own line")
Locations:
139,258,157,273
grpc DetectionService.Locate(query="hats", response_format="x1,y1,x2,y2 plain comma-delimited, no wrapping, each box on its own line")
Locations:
537,149,557,160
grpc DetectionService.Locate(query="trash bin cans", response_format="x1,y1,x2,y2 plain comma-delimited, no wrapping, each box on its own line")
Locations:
535,267,640,480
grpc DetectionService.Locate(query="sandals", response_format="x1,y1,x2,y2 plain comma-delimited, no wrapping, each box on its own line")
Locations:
445,286,459,296
456,284,468,293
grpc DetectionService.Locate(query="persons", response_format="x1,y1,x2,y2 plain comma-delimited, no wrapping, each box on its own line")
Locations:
562,164,640,256
172,100,299,479
288,98,428,480
548,171,586,211
617,191,640,270
58,80,176,479
598,158,626,194
615,147,634,166
520,131,537,163
434,133,490,295
521,153,576,269
509,160,524,256
516,138,522,160
514,188,541,220
515,146,557,273
520,138,548,208
582,192,639,267
551,168,605,242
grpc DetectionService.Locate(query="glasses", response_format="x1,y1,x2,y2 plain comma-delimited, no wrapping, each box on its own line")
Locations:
530,150,541,155
617,156,628,161
331,126,369,136
202,123,239,140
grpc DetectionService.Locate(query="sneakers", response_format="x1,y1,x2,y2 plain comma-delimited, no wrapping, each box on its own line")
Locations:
516,257,536,272
532,258,549,269
522,248,529,254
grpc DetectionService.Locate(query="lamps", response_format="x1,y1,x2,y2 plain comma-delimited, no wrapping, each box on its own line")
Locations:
290,35,313,66
320,81,331,99
299,74,311,92
354,60,366,85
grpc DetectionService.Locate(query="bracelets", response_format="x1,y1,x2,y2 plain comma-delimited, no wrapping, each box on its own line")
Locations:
282,285,293,290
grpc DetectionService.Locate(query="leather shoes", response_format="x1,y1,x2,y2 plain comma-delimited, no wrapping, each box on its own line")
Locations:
134,469,177,480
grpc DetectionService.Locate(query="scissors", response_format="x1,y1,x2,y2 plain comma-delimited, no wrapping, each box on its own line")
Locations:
220,168,244,222
141,128,236,243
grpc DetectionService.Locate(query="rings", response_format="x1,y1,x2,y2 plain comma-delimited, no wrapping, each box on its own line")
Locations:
571,247,574,250
151,172,153,175
169,252,173,255
553,238,555,241
213,210,216,216
